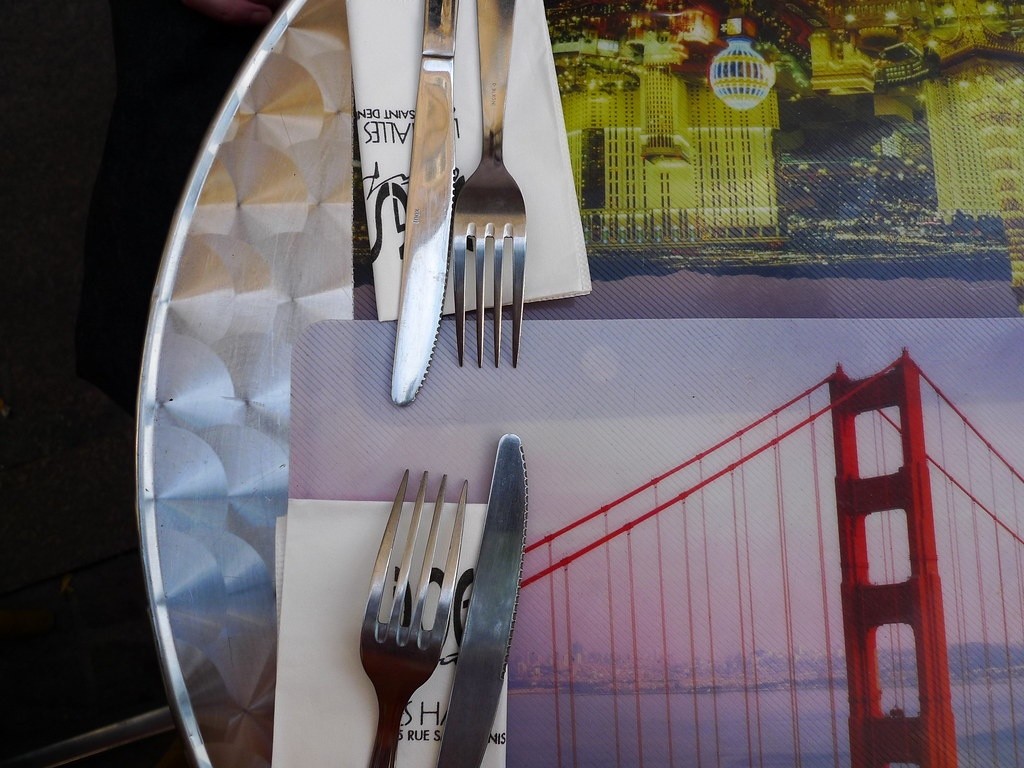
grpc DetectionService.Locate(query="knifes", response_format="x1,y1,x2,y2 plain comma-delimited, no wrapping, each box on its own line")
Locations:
390,0,460,406
437,432,529,768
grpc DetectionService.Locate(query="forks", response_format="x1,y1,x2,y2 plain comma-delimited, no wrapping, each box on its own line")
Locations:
451,0,529,370
359,469,470,768
